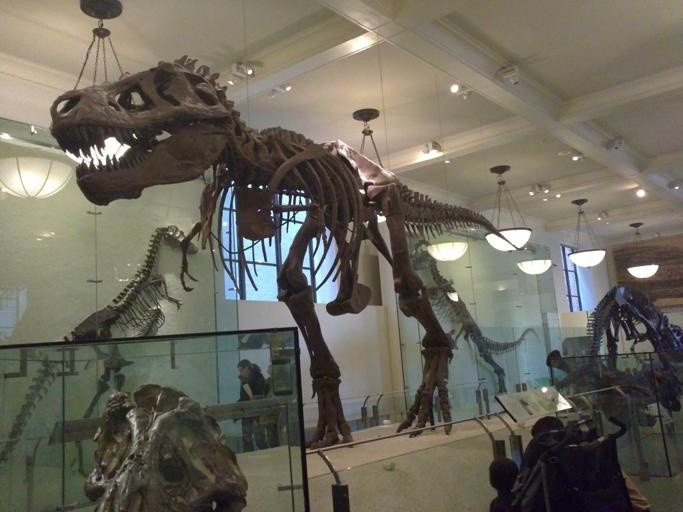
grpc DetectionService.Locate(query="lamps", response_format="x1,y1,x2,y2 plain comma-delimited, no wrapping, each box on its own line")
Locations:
271,359,295,512
427,223,469,261
0,118,74,200
63,1,140,168
543,191,561,202
484,166,531,252
267,82,291,100
516,244,552,276
353,108,390,224
225,62,255,86
568,199,606,268
628,223,659,279
597,210,610,225
529,182,550,196
451,83,471,100
422,138,442,158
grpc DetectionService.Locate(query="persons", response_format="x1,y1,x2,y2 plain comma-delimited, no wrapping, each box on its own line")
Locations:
261,365,281,449
530,416,651,510
488,456,521,512
233,359,268,452
